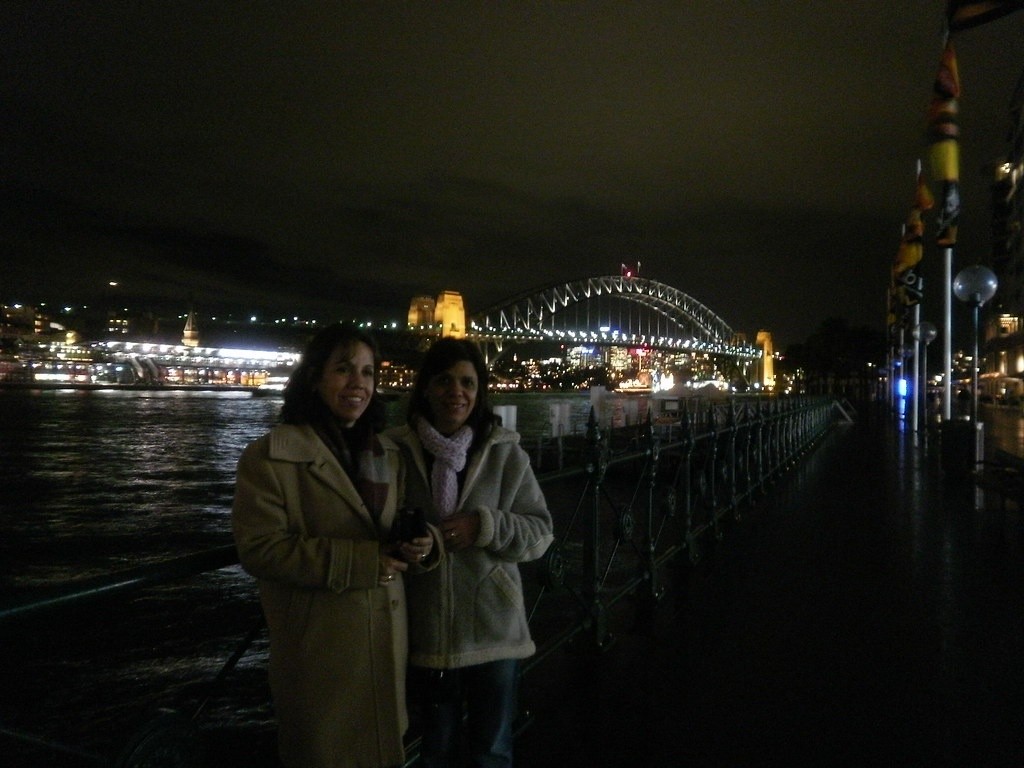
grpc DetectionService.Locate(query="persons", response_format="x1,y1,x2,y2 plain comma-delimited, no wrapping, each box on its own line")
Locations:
231,322,554,768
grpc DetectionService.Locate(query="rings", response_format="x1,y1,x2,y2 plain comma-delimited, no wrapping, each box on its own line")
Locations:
451,529,456,536
421,553,426,560
385,575,391,582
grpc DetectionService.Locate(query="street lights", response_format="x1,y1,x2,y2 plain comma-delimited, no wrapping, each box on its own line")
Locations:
951,264,999,514
911,322,938,471
